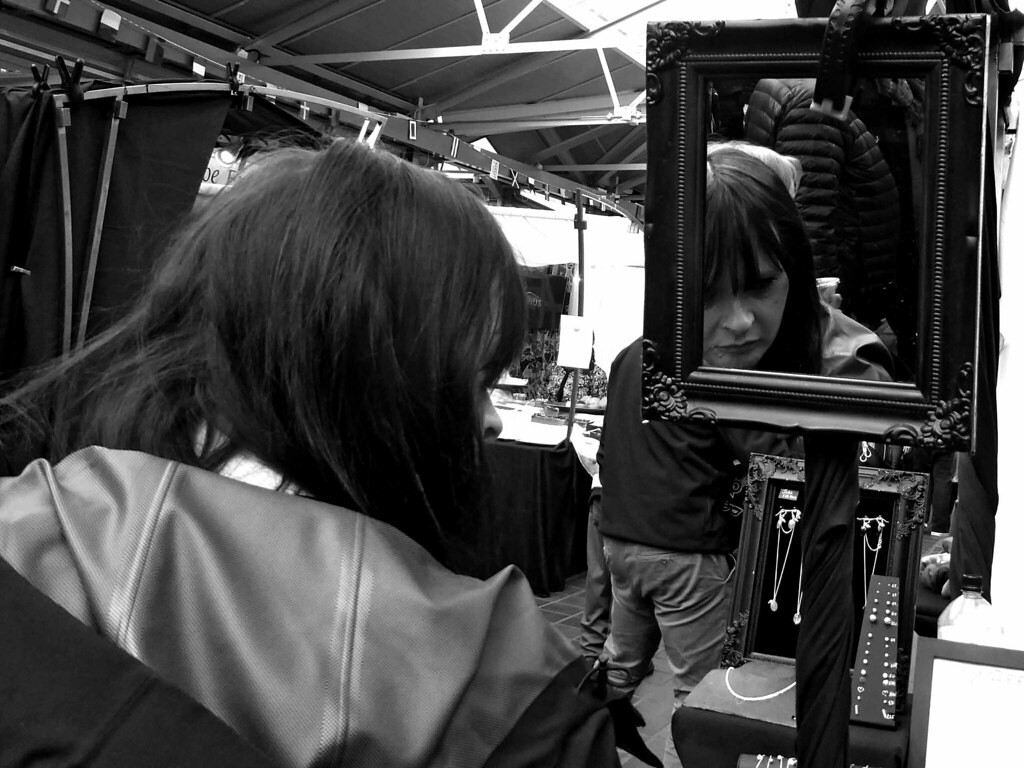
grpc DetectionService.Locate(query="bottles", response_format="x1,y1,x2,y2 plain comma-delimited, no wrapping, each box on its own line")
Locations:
937,572,993,646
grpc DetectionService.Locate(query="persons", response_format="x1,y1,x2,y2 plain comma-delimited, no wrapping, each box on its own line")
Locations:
582,475,611,662
0,138,621,768
598,340,786,768
703,80,926,386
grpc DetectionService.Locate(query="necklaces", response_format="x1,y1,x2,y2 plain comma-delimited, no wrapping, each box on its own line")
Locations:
724,501,887,704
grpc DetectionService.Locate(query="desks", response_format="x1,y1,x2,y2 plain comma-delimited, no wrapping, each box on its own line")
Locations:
484,416,602,598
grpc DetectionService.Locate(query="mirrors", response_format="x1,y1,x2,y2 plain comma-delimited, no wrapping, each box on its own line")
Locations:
644,13,990,454
